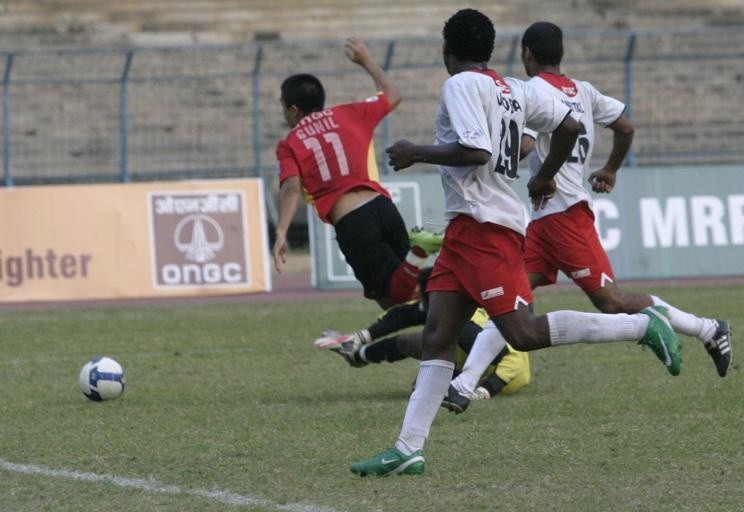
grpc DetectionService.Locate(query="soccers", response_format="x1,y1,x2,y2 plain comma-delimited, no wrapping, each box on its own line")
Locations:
79,355,125,401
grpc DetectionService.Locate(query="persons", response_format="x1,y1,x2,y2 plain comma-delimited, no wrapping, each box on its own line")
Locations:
273,35,445,311
312,267,531,402
412,22,732,412
351,8,680,476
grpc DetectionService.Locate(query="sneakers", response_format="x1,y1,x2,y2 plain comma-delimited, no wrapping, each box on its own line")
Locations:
408,226,445,255
703,319,734,378
312,327,374,368
350,447,426,475
442,383,471,416
637,305,683,376
